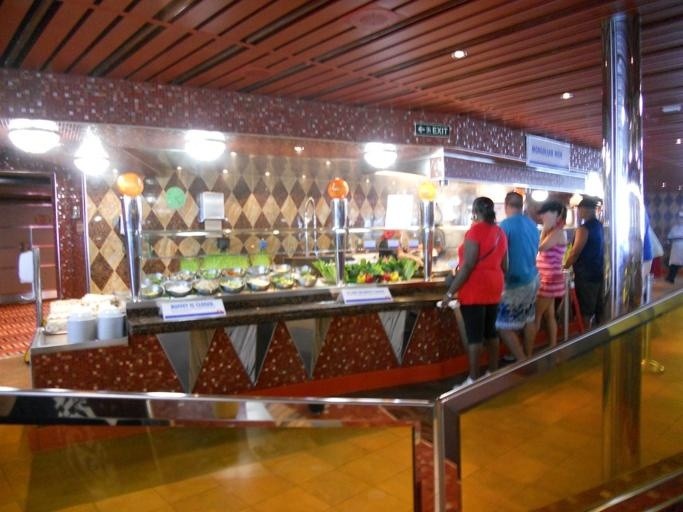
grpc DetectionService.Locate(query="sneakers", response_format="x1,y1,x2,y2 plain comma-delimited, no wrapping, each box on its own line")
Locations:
498,354,515,363
438,376,473,399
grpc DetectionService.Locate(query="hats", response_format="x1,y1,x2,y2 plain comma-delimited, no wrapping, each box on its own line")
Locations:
575,198,595,208
536,200,561,214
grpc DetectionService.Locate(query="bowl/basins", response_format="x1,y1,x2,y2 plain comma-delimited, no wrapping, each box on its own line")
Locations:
140,263,318,300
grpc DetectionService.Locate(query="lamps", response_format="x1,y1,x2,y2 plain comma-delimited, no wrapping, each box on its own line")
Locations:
74,157,108,176
8,129,61,155
531,190,548,203
182,140,224,160
364,152,397,168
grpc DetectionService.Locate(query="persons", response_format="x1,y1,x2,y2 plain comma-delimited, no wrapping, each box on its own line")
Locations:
377,230,426,268
562,196,606,335
438,198,510,391
523,198,570,360
493,191,542,366
663,210,683,284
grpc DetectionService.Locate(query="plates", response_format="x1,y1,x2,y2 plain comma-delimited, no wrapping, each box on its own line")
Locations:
64,309,126,347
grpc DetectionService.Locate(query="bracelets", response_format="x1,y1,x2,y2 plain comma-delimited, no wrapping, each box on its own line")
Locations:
445,290,453,298
